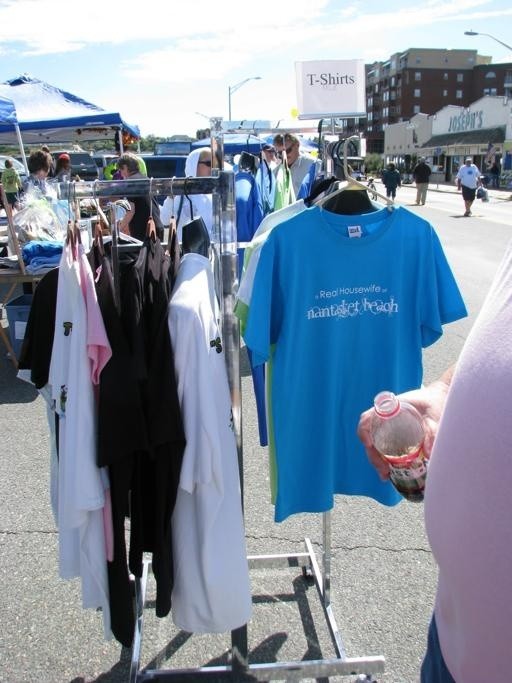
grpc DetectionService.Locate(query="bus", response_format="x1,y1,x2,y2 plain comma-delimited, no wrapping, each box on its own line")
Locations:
334,156,367,180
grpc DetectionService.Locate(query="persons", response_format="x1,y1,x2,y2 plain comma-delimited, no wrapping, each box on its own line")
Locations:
490,156,501,188
19,147,57,202
1,157,22,207
262,142,278,170
116,152,164,241
381,160,401,205
357,244,510,683
274,131,320,199
159,146,212,243
412,156,431,205
417,158,420,163
456,156,489,216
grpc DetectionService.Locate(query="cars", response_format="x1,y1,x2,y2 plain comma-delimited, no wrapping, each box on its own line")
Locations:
0,147,190,199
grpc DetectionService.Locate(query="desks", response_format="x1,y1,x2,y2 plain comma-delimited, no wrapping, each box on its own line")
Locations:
0,268,48,371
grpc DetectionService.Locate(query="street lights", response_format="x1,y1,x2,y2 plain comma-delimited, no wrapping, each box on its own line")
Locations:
228,74,265,123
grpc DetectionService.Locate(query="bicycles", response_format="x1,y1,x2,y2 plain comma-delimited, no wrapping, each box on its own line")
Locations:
363,177,380,201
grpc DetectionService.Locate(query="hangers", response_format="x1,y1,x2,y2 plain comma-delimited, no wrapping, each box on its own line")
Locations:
304,135,394,210
66,175,210,258
238,120,288,174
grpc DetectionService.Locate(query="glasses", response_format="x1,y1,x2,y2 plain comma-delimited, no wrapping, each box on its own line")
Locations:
277,144,293,156
198,160,211,166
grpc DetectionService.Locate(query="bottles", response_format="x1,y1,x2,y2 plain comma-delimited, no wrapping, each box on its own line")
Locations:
371,390,425,502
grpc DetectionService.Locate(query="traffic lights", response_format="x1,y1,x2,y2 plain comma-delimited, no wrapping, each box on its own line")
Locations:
464,30,512,54
